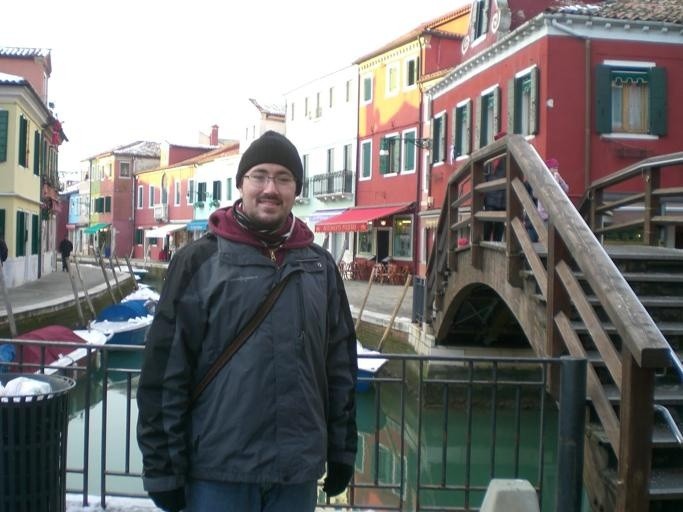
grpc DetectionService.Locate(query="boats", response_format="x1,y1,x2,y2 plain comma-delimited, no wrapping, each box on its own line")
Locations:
353,390,388,439
357,338,390,396
112,263,150,280
105,348,146,386
135,275,142,283
0,324,115,395
91,285,162,354
60,379,103,422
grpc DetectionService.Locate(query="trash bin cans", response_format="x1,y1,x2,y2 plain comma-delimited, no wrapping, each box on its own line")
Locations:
0,373,76,512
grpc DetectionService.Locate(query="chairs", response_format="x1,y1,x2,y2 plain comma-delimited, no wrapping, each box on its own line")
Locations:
332,257,410,286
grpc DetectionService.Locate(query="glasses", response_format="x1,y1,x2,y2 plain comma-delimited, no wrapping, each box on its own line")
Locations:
238,171,296,186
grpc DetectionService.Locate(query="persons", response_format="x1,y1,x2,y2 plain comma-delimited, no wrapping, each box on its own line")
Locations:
137,129,358,512
482,131,511,241
537,158,569,241
523,179,539,242
59,235,73,272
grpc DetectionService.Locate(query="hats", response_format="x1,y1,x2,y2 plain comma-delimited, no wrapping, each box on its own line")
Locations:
544,158,559,170
234,128,304,199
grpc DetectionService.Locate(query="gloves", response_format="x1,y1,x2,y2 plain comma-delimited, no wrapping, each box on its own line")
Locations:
318,460,352,499
147,485,186,512
